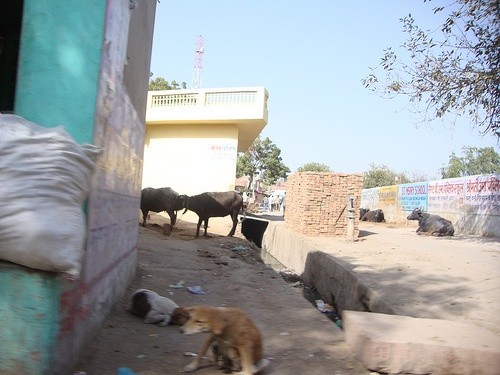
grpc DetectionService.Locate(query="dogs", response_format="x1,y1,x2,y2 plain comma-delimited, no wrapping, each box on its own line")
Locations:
177,306,269,375
127,288,182,327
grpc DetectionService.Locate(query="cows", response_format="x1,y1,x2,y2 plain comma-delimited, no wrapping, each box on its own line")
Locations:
359,208,370,220
140,186,246,238
361,208,384,223
407,207,455,236
